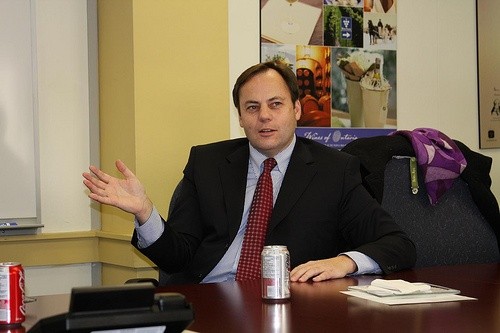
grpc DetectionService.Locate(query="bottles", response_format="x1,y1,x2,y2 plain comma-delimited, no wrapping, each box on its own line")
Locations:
371,54,384,87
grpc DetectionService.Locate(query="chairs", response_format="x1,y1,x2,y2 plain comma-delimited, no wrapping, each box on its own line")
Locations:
122,156,499,287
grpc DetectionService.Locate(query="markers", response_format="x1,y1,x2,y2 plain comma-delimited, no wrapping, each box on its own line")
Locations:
0,222,17,227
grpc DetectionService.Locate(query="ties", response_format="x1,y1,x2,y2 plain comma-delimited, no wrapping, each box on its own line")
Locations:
235,158,277,281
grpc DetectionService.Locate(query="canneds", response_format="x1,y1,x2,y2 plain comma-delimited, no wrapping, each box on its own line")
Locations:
0,262,26,326
261,245,290,300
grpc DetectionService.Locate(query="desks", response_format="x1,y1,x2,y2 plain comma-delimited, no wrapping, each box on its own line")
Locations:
0,264,500,333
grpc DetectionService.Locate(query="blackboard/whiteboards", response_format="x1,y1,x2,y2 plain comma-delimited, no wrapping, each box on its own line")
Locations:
0,0,101,229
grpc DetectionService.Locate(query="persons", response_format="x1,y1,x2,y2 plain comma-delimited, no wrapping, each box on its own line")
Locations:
82,60,418,286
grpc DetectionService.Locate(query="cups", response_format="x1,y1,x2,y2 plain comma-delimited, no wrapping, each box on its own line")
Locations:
344,74,390,128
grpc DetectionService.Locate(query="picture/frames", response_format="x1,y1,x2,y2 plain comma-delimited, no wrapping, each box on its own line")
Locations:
475,0,500,149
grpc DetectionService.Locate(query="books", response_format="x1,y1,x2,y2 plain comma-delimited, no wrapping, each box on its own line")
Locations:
346,282,460,300
